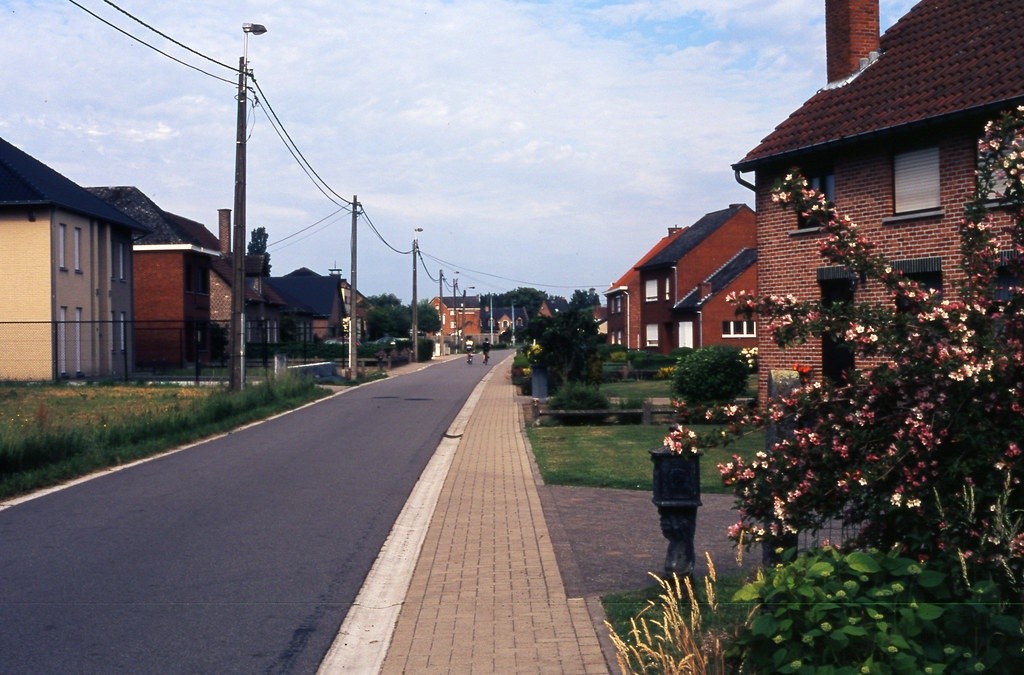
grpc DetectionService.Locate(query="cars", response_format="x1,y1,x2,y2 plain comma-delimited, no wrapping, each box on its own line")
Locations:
325,337,362,347
375,336,402,345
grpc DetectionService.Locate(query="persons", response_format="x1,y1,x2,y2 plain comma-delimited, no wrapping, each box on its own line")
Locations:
465,336,474,362
482,337,490,364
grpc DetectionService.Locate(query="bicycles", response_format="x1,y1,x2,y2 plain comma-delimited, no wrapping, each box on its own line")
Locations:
466,349,473,364
483,351,490,365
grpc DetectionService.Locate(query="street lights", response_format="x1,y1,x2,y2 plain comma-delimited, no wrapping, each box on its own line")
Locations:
410,227,424,362
452,270,459,351
229,20,266,398
